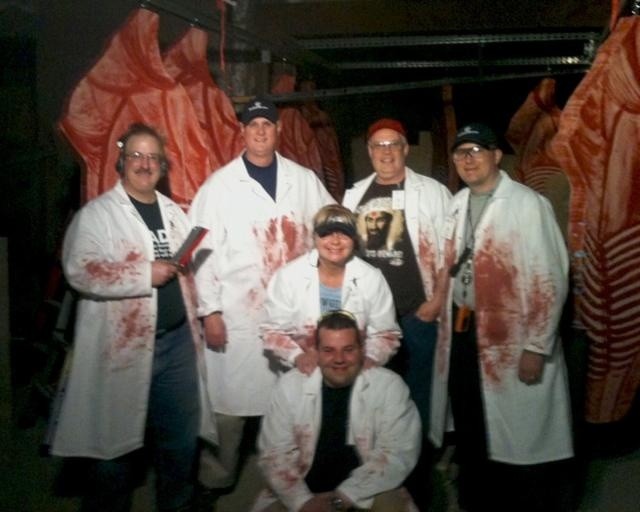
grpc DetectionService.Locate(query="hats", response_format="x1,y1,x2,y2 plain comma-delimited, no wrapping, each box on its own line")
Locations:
451,122,498,151
241,99,278,124
366,119,404,137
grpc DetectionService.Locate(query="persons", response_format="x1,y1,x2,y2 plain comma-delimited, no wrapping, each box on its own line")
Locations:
254,204,405,377
362,198,393,251
187,100,340,505
341,119,454,466
254,309,424,512
50,123,220,512
443,122,573,511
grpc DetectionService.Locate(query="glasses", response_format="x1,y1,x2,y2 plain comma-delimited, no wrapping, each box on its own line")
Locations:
125,152,160,161
454,147,482,162
369,141,404,151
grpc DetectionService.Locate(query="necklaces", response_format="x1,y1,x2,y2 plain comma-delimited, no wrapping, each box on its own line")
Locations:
461,191,493,285
